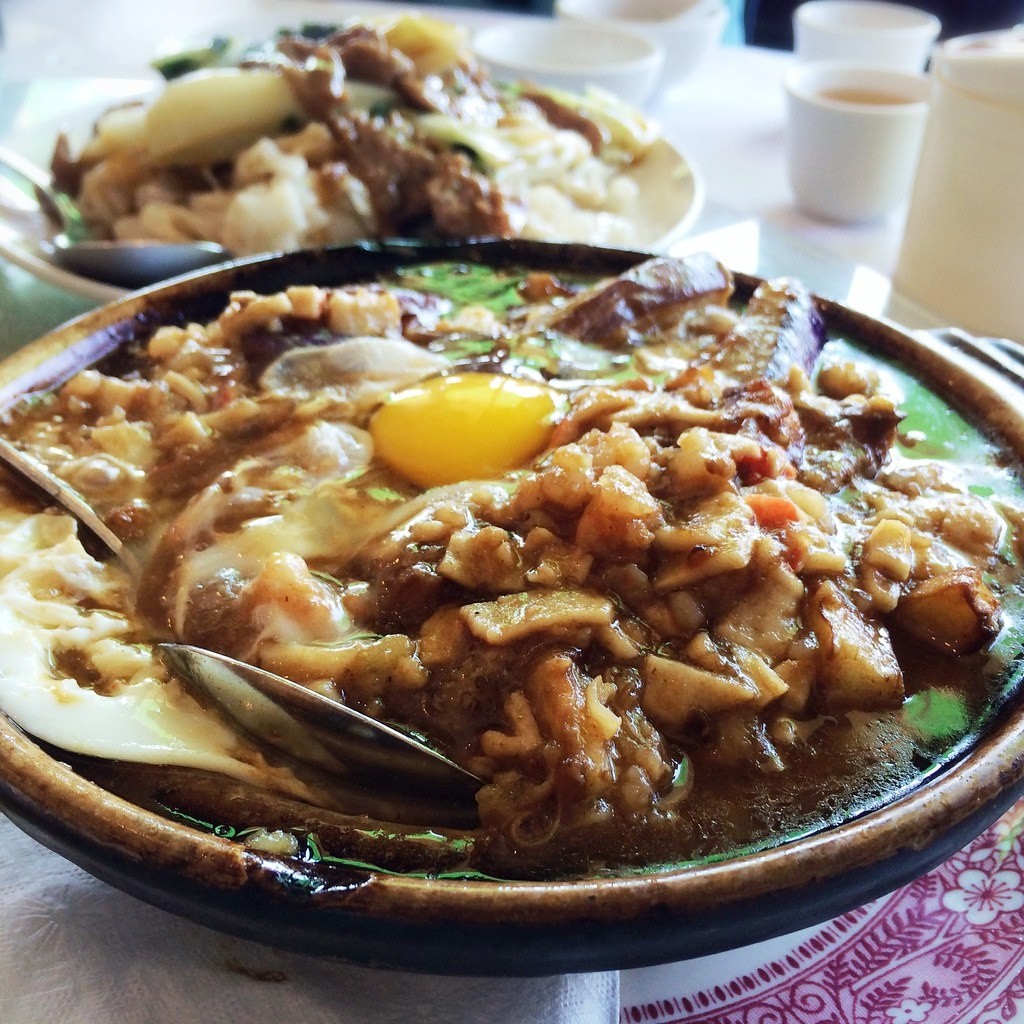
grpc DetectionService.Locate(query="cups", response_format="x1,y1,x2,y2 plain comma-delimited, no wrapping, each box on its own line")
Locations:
892,24,1023,346
782,55,931,221
550,0,728,92
792,0,940,74
472,16,660,111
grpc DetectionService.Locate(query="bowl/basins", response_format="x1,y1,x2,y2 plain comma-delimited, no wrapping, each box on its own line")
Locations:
1,86,702,299
0,240,1024,977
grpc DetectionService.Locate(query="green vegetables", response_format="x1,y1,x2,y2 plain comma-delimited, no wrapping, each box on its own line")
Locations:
362,263,1024,737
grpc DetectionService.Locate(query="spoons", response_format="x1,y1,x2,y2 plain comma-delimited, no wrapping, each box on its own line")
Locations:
1,151,230,281
1,440,489,804
